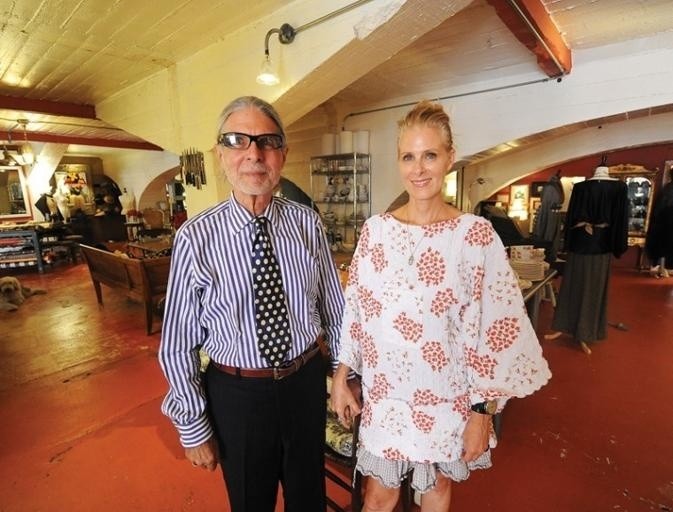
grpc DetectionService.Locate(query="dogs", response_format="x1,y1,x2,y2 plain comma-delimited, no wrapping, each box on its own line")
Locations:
0,276,46,312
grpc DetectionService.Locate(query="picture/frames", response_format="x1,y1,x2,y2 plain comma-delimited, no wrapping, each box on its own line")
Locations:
51,164,93,202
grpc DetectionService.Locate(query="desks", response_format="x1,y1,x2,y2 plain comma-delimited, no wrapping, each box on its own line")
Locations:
519,269,558,336
127,238,175,256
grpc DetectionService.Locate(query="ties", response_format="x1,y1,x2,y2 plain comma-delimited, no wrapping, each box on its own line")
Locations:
251,216,292,367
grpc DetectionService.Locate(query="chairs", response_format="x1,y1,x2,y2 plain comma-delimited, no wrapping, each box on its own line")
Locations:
142,209,165,229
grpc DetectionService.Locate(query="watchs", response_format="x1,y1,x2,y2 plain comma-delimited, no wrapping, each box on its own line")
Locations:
471,400,497,415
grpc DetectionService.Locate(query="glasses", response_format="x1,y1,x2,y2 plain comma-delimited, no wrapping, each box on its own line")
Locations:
216,131,286,151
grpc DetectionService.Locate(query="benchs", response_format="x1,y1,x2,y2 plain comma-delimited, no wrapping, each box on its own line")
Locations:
78,242,172,336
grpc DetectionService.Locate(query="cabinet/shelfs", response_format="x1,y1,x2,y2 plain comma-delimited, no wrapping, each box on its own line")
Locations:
0,231,43,273
607,164,658,248
309,152,371,255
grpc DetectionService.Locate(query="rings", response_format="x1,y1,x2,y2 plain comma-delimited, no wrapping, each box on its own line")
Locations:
338,418,344,421
192,462,197,467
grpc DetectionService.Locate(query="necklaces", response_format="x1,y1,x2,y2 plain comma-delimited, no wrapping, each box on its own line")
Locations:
406,204,443,265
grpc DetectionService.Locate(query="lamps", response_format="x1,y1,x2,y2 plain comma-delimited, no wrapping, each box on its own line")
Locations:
16,118,33,165
256,24,295,82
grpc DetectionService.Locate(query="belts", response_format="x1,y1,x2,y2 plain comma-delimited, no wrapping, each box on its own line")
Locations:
209,344,320,381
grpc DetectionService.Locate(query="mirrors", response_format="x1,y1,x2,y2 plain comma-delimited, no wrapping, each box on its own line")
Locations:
0,165,31,218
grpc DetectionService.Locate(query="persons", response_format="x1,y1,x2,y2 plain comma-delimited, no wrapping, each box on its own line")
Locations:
535,169,564,261
157,95,361,512
330,102,553,512
551,156,628,342
